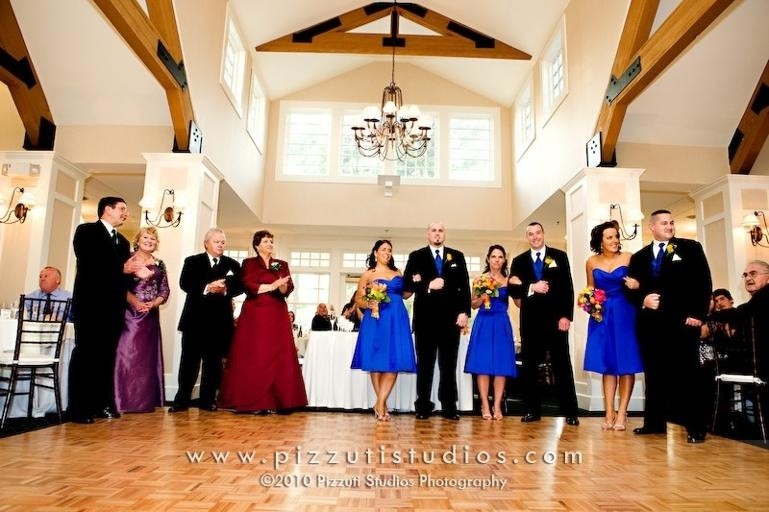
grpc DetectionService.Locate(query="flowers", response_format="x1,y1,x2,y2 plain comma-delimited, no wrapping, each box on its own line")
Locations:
362,283,391,319
667,244,677,255
272,262,279,270
472,275,503,309
544,256,553,265
577,287,607,323
446,254,454,261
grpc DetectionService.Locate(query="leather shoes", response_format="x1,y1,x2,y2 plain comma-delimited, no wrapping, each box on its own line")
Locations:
168,404,181,413
103,408,118,418
687,429,706,443
633,426,660,435
524,413,540,422
446,412,460,420
205,403,217,412
415,412,428,420
567,416,579,426
75,415,94,424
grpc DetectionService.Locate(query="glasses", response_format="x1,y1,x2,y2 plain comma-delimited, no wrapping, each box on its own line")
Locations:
741,271,768,277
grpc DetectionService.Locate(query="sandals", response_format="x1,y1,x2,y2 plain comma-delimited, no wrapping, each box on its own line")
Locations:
613,413,627,431
492,408,503,420
481,406,493,421
601,413,617,429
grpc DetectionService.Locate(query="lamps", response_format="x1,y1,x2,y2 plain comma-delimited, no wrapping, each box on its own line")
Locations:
352,1,431,161
0,188,34,224
609,204,644,241
742,211,769,248
140,189,184,227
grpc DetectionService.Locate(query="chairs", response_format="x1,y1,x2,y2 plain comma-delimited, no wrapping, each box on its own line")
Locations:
708,315,769,444
0,295,72,428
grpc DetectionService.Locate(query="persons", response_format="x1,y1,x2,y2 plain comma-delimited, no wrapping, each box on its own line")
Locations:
464,244,523,420
354,239,421,422
67,194,142,423
168,229,243,413
698,260,769,378
19,265,73,322
624,209,711,443
583,221,639,431
114,226,171,415
217,230,307,415
508,222,579,426
230,292,365,337
402,222,471,420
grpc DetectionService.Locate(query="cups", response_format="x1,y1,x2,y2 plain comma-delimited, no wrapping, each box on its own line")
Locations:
0,300,39,321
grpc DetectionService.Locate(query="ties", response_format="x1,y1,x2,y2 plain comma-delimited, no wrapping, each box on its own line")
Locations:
435,250,443,275
213,257,218,267
534,252,544,281
43,293,53,315
655,243,666,268
112,229,118,243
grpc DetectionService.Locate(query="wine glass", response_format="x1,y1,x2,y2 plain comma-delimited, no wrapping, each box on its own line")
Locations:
328,311,355,332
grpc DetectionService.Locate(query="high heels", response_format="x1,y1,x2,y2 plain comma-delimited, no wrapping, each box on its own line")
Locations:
373,405,391,422
